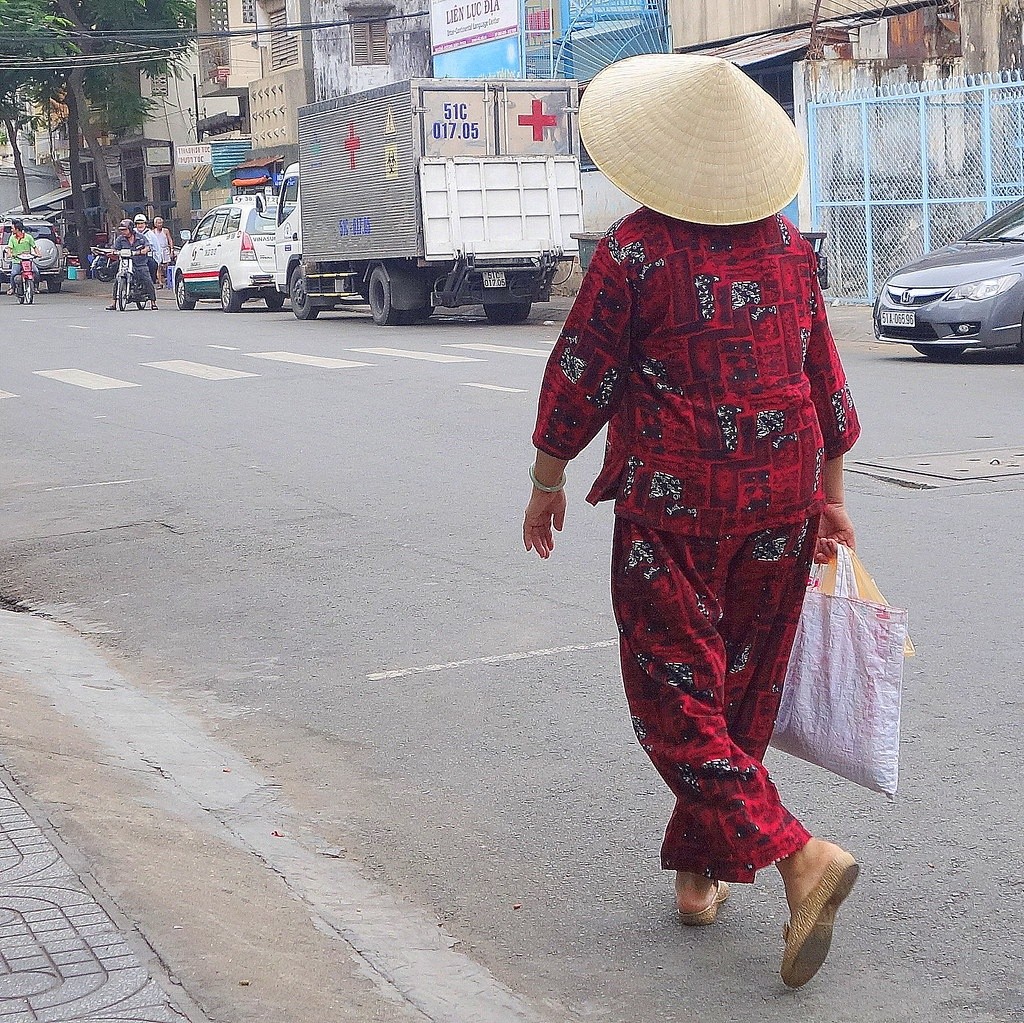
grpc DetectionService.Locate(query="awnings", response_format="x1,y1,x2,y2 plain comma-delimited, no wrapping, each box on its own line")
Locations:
685,15,901,70
5,180,177,220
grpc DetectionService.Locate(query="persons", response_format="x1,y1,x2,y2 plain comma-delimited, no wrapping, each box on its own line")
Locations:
105,219,158,311
131,214,158,284
5,219,42,295
519,53,864,988
151,216,174,290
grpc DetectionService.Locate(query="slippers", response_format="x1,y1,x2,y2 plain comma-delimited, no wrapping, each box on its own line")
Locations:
677,880,729,926
781,852,860,988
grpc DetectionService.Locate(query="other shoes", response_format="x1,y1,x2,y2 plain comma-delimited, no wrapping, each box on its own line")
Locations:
156,284,163,290
151,304,158,310
34,289,40,294
106,304,116,310
7,288,15,295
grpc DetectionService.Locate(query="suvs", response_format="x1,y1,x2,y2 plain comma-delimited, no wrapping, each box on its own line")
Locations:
0,214,65,293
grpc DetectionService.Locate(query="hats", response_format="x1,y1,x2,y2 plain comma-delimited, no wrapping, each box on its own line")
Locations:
578,52,806,227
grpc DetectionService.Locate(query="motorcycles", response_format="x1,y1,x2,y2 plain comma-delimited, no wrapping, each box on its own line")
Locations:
8,251,42,305
90,245,122,283
108,238,146,312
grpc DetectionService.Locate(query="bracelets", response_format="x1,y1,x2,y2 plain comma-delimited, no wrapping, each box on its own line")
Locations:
171,249,174,252
529,461,567,492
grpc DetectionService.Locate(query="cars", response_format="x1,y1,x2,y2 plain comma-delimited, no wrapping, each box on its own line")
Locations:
872,196,1024,363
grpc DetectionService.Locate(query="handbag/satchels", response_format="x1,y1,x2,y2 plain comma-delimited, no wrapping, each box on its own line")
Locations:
770,538,914,802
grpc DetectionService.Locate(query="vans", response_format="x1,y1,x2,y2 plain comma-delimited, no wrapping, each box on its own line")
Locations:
172,201,299,315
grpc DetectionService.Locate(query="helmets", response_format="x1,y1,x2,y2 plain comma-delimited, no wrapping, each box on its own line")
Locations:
134,214,146,223
118,219,134,232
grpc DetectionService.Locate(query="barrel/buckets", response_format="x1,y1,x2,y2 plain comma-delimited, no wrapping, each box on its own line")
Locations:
67,266,76,280
76,269,88,281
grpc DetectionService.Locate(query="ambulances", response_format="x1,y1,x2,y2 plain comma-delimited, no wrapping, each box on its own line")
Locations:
254,75,580,328
